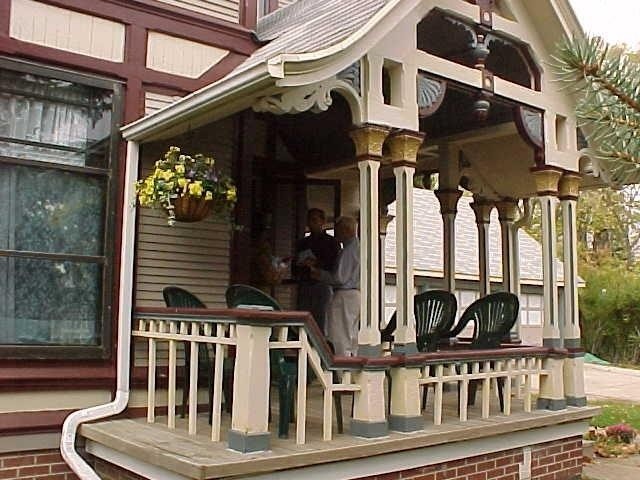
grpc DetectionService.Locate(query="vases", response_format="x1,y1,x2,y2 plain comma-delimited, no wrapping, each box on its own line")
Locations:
160,195,212,221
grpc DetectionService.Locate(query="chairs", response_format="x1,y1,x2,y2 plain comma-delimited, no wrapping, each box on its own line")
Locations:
157,281,233,425
379,285,458,418
418,289,517,416
226,283,346,443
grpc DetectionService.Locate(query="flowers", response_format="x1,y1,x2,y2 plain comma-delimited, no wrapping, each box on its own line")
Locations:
131,144,245,236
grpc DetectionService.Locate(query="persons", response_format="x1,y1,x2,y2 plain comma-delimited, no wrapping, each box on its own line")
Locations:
291,209,338,336
307,216,360,383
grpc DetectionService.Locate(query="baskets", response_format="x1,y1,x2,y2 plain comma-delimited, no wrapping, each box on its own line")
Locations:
165,195,216,222
